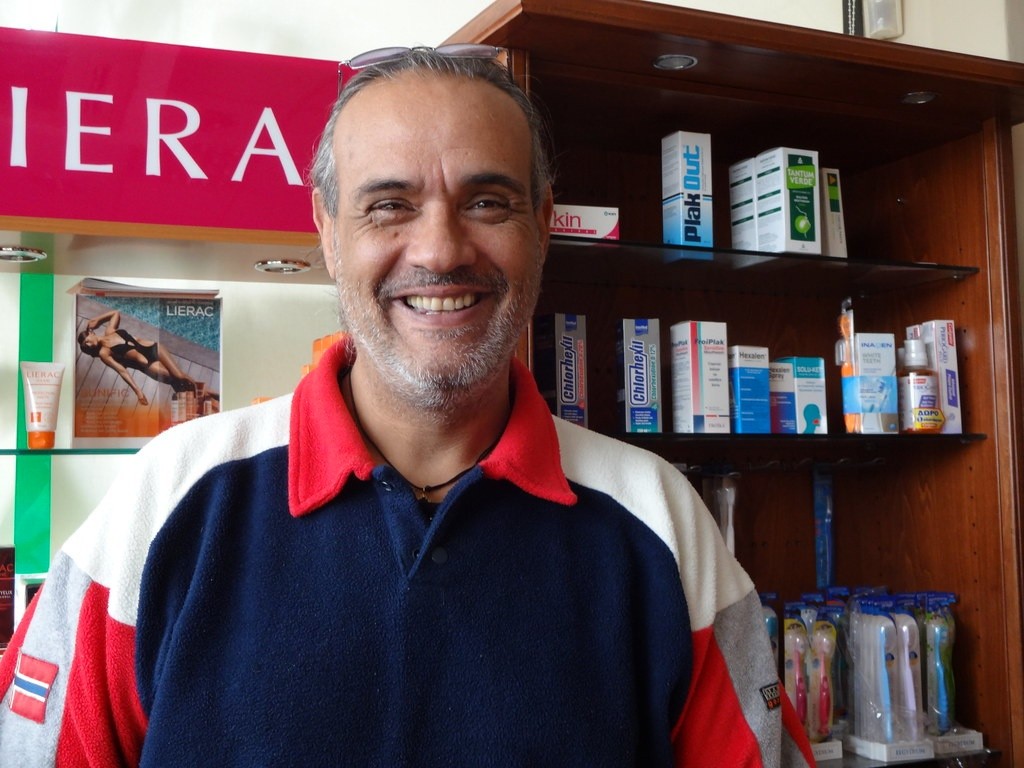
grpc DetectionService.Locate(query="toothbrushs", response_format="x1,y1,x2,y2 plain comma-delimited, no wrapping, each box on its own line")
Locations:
755,582,957,744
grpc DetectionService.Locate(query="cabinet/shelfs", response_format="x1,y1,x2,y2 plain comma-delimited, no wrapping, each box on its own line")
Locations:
435,3,1024,768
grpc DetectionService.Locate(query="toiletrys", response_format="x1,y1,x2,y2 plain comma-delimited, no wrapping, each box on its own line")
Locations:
19,361,66,449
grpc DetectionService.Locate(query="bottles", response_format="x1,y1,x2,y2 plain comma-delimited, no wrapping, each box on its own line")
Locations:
899,339,941,434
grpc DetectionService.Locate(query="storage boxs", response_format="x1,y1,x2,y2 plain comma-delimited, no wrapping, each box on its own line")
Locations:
661,132,714,260
856,315,962,434
669,321,731,433
822,168,847,262
729,346,771,433
729,158,759,270
553,311,586,431
622,318,662,433
756,146,821,264
768,356,828,432
548,204,620,244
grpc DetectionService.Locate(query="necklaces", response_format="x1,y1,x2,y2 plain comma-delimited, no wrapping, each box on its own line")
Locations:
347,359,516,527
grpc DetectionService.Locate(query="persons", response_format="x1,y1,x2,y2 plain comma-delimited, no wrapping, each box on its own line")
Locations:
0,47,782,768
78,310,218,414
